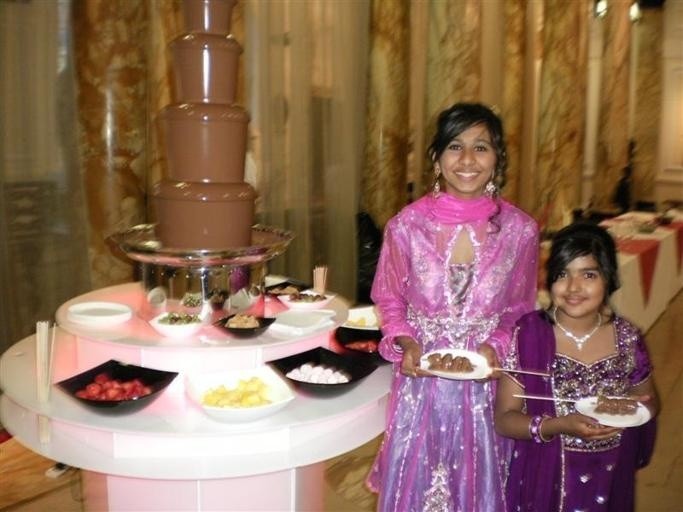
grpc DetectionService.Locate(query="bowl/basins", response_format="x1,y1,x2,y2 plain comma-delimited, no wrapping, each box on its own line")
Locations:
652,216,674,227
210,313,276,339
144,284,263,312
632,220,657,233
277,289,336,310
265,346,379,399
148,312,202,337
183,369,298,422
51,358,179,420
330,325,395,367
265,281,313,301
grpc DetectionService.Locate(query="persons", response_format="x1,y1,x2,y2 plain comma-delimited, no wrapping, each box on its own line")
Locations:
364,101,540,512
494,222,660,512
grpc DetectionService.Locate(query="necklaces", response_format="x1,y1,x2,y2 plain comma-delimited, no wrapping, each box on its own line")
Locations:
552,308,603,351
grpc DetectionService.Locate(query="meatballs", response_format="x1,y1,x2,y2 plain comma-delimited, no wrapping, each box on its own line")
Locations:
286,363,349,385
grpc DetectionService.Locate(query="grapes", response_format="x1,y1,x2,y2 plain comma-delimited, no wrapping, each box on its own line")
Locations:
184,296,202,307
158,313,200,325
287,293,326,303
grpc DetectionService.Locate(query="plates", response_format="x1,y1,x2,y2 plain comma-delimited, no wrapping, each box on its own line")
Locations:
67,302,132,325
341,305,382,331
572,396,654,430
419,346,493,381
270,308,336,336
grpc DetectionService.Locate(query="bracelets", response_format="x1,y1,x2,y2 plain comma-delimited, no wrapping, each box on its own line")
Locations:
528,415,556,445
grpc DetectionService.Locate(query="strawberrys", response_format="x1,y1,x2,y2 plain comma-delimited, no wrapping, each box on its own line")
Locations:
73,374,153,400
